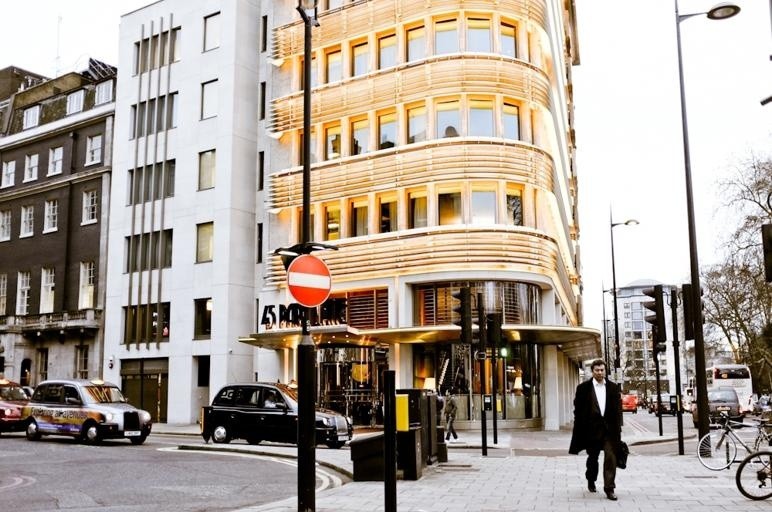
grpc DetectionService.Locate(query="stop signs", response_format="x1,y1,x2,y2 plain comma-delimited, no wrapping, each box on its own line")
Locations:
284,255,333,309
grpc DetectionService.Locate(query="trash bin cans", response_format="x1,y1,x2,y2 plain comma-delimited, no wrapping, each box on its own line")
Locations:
348,431,386,482
401,427,422,480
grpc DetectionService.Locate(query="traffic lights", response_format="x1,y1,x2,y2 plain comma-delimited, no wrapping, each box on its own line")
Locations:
642,283,666,343
453,285,473,346
498,336,512,360
652,325,666,356
680,283,706,342
469,291,486,349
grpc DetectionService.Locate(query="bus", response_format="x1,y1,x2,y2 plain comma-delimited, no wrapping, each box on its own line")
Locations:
686,363,753,416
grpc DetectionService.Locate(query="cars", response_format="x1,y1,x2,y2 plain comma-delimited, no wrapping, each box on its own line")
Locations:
691,388,744,429
1,371,153,446
621,386,697,417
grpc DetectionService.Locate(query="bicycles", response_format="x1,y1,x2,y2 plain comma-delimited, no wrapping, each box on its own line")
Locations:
737,449,772,502
697,407,772,473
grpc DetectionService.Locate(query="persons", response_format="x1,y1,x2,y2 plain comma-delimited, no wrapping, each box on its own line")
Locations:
444,389,459,444
433,391,443,426
568,360,624,501
748,391,772,415
265,392,278,409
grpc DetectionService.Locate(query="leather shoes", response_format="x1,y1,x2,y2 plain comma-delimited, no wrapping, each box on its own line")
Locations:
588,479,597,492
603,486,618,500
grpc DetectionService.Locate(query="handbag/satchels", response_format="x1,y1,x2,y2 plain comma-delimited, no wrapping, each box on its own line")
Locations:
614,439,630,469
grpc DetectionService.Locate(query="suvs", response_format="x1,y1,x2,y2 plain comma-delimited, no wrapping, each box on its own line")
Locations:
204,381,353,451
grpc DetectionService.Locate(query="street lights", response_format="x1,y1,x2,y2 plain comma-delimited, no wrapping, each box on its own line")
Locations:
600,282,620,377
673,3,744,457
609,205,640,379
